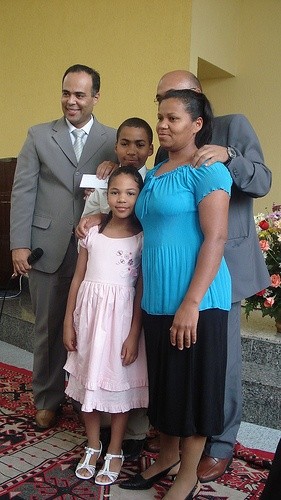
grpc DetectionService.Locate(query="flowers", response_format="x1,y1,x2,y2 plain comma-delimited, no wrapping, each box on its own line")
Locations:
244,202,281,321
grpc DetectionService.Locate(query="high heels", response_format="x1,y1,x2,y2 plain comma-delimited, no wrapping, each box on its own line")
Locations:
185,480,202,500
119,459,181,490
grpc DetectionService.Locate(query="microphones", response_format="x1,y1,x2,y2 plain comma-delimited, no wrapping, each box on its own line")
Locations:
11,248,43,279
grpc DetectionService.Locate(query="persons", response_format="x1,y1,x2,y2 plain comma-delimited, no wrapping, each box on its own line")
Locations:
9,64,120,431
158,71,273,483
75,88,234,500
61,164,145,485
82,117,155,459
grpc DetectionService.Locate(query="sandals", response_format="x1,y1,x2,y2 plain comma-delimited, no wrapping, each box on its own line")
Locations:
75,441,102,479
95,449,125,484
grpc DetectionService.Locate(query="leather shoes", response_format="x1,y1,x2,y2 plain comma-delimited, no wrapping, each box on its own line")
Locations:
123,439,144,461
78,411,86,423
35,409,56,427
146,436,160,452
100,428,112,448
196,454,232,482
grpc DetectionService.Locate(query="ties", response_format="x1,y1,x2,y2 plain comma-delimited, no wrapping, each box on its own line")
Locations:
71,129,86,164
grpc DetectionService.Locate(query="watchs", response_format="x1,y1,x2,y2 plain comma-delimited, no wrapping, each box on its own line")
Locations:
223,146,236,165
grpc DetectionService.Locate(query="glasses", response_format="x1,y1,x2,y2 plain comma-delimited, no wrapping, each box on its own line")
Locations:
153,96,163,105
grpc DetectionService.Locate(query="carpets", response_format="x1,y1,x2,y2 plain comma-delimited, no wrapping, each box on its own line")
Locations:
0,362,274,500
